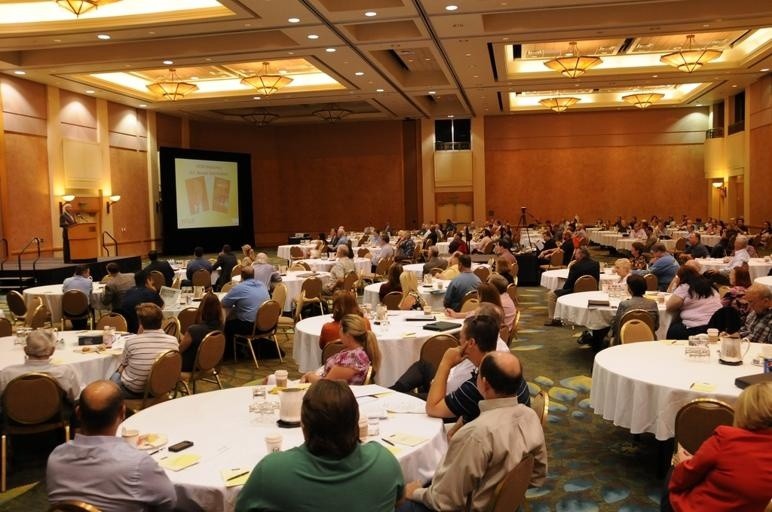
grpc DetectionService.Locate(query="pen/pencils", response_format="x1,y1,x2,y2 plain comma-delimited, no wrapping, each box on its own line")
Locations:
407,331,416,337
670,340,678,344
146,445,167,456
367,394,379,398
382,437,395,448
227,469,250,482
688,381,697,390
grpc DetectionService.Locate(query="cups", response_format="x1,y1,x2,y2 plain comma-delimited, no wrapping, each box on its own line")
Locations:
368,417,380,436
262,409,274,427
264,437,283,453
253,387,266,403
25,327,33,337
707,328,719,344
358,415,368,438
321,253,327,260
121,430,139,446
16,328,25,344
249,403,263,423
275,370,288,387
423,306,432,315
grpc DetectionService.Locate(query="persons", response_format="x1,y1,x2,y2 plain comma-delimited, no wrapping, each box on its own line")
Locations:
47,380,178,512
63,214,772,420
396,351,548,512
661,382,772,510
234,379,405,512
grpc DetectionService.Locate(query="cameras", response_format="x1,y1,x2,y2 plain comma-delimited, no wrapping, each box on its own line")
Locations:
520,207,528,215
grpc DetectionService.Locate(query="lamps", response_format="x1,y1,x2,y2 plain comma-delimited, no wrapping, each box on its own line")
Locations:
543,42,604,79
241,63,293,96
59,195,77,212
621,93,665,114
241,108,279,128
55,1,122,17
145,68,199,103
712,181,727,198
659,34,723,73
538,92,581,115
105,195,121,215
311,103,352,129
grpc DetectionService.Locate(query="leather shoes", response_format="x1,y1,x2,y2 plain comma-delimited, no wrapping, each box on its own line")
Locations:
543,320,562,328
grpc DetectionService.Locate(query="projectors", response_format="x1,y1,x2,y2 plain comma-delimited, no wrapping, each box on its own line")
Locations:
295,233,310,238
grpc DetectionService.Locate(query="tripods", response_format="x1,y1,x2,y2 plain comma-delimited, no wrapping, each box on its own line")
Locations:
512,215,532,249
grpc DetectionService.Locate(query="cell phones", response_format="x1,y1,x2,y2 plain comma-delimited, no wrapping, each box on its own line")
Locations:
168,440,193,452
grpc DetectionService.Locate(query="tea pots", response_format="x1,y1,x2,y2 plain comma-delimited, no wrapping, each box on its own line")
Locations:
328,251,337,261
192,284,205,301
718,335,751,366
277,388,303,427
375,302,388,320
278,258,289,276
422,271,432,287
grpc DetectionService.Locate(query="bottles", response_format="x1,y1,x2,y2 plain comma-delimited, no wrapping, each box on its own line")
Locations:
103,325,112,349
